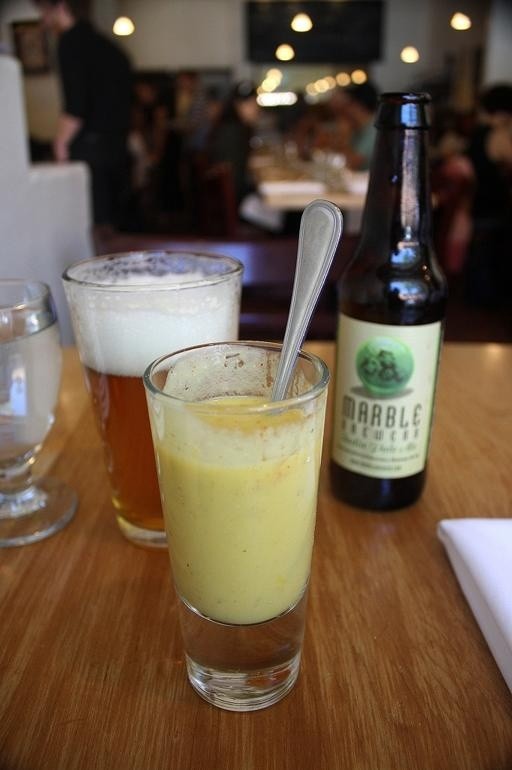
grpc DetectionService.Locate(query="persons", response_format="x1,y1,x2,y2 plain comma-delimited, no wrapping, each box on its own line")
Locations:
136,73,381,228
39,0,137,227
457,82,512,258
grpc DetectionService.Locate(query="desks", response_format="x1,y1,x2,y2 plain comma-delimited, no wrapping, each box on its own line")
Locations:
0,342,512,770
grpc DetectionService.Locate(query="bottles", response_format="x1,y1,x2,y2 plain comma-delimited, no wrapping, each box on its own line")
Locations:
332,90,453,512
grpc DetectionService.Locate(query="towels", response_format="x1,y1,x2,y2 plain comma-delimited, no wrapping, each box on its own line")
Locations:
437,518,512,695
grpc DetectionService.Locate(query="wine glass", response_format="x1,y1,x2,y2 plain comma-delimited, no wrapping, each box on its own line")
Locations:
0,281,80,546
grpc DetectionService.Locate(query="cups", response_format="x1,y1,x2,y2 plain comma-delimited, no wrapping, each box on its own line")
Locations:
59,250,245,550
142,341,330,714
